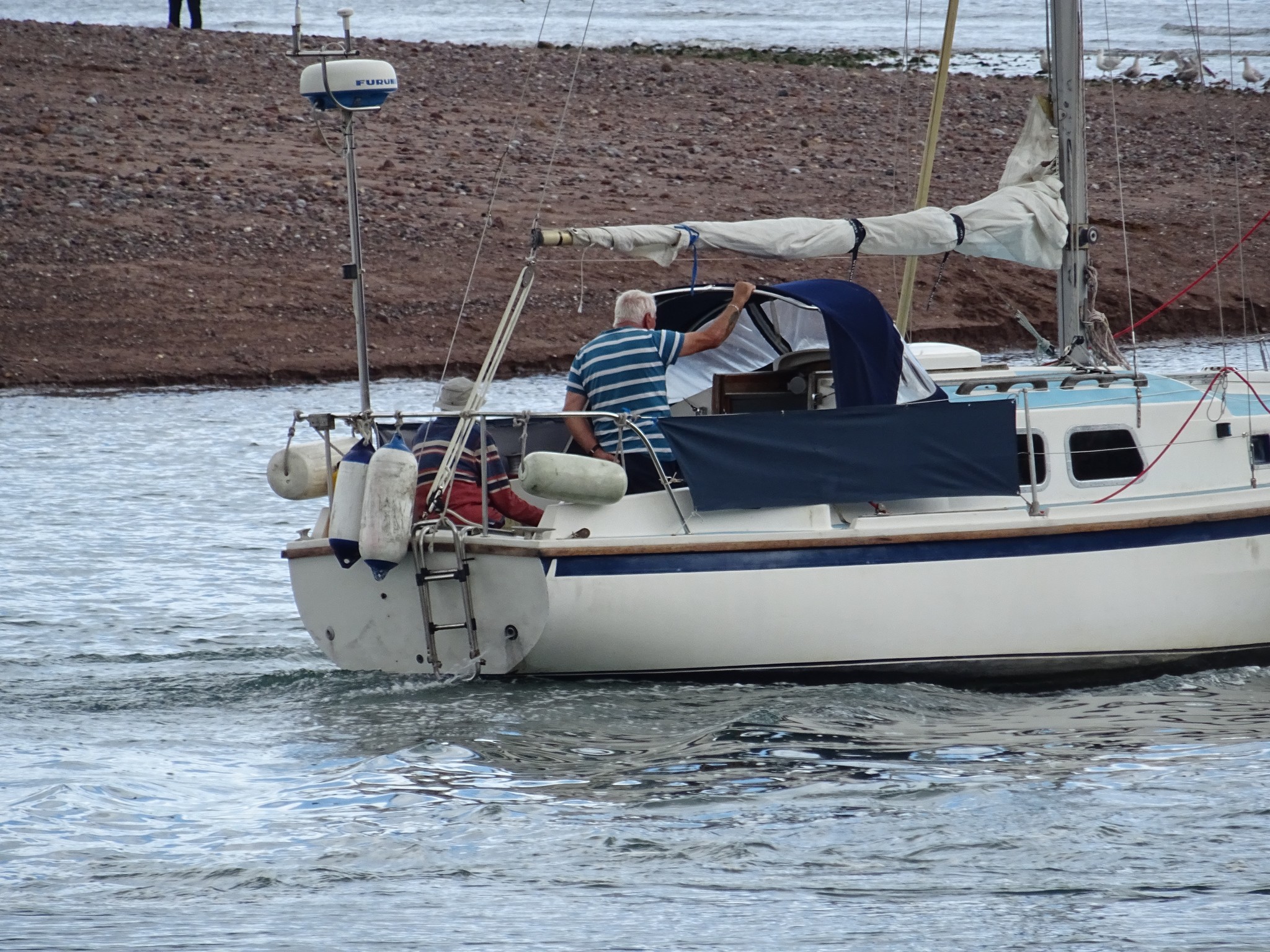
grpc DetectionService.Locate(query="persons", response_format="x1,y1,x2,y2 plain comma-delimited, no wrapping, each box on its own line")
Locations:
562,280,755,495
167,0,202,30
411,376,544,528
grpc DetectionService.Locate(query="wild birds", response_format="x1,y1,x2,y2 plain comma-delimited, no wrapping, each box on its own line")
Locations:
1033,48,1053,76
1094,48,1128,78
1116,53,1145,81
1146,46,1217,89
1238,56,1270,92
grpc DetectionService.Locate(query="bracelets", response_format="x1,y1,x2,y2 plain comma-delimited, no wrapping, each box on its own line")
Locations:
727,304,741,318
589,443,599,457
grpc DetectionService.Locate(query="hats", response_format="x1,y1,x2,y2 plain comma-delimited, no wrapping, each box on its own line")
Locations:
434,377,487,412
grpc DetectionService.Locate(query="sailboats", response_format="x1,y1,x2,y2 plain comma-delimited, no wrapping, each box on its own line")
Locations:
267,0,1269,690
